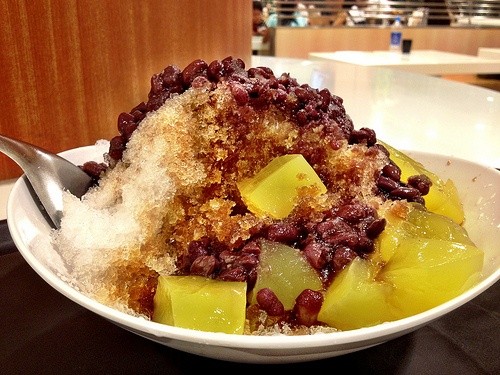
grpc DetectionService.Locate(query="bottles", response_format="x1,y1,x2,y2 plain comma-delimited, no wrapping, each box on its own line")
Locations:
389,17,403,51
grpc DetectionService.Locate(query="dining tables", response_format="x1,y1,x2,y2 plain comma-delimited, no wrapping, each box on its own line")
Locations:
0,51,500,375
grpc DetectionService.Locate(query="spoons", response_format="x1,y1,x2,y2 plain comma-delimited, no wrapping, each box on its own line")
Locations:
0,135,97,228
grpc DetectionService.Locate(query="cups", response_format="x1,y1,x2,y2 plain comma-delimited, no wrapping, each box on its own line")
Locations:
403,39,411,53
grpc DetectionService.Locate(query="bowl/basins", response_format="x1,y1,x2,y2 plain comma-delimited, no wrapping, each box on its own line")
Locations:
8,144,500,362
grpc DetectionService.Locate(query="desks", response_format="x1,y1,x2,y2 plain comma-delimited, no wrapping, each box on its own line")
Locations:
309,49,500,78
